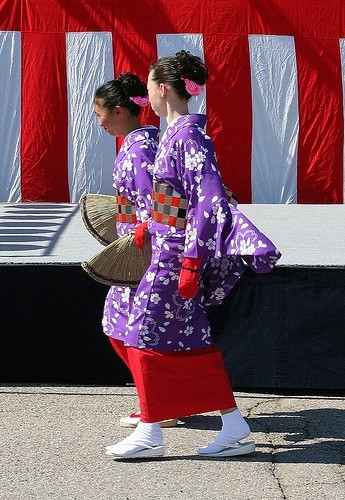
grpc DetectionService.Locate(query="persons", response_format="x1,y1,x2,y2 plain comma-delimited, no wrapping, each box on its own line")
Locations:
94,71,178,427
103,49,283,460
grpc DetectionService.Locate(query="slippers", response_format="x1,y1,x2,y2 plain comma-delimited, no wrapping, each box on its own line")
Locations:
200,436,256,457
119,410,178,428
112,440,165,460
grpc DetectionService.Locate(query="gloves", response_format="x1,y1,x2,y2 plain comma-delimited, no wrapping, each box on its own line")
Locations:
134,222,148,248
178,257,203,300
